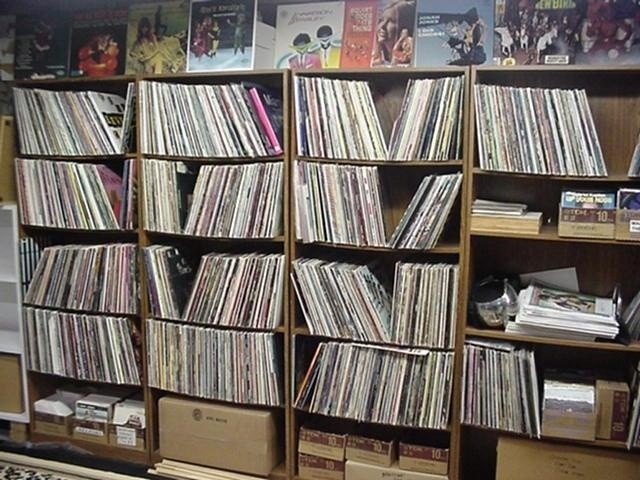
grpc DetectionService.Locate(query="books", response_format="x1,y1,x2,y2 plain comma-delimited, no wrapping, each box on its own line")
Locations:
559,186,640,241
540,356,640,452
458,336,543,440
34,380,146,450
20,236,145,386
2,1,639,83
290,254,460,430
292,159,464,250
14,157,139,232
142,243,286,409
141,158,285,239
627,140,639,178
470,198,543,237
140,80,284,158
504,269,639,345
474,83,608,177
11,82,137,156
291,74,464,162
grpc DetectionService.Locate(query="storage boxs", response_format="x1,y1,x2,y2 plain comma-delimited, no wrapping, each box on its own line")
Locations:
556,188,613,238
493,437,639,480
108,397,146,449
33,390,74,437
295,453,343,479
597,376,633,439
72,394,122,444
342,461,448,479
344,434,398,467
615,188,640,241
298,423,347,461
157,396,278,477
542,373,597,440
398,442,449,473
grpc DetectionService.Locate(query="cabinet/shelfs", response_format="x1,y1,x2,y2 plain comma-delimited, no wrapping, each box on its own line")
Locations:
2,73,140,468
456,63,640,480
292,66,470,479
141,68,292,478
